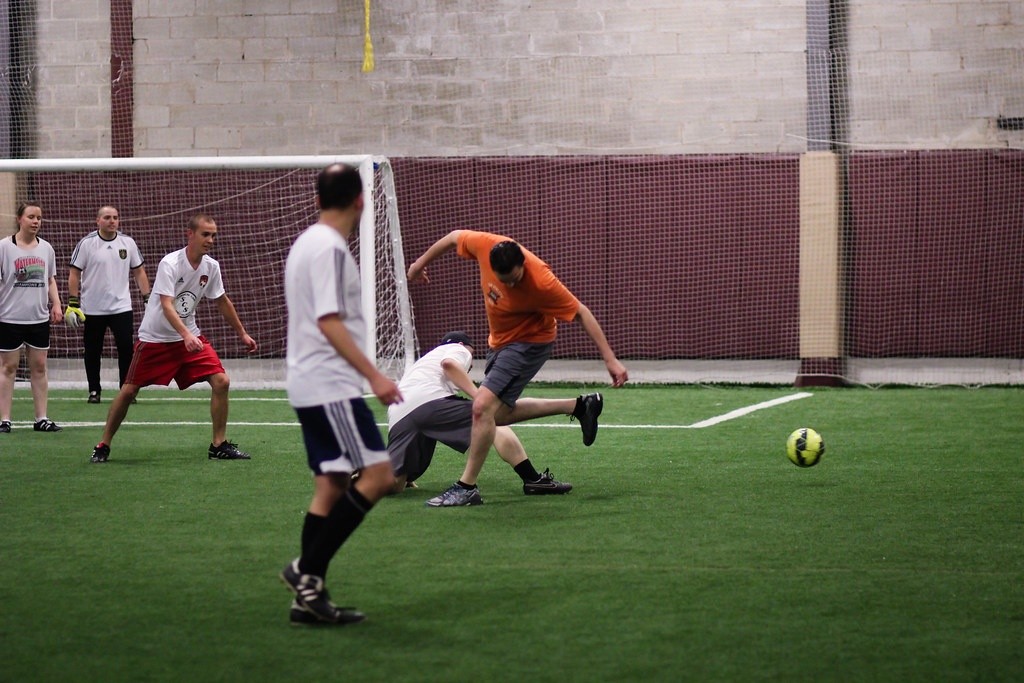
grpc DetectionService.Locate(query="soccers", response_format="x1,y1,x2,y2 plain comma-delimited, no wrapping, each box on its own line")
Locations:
787,427,825,466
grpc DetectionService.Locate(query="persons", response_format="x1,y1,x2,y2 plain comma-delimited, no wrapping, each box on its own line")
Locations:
408,226,628,508
276,160,406,627
64,205,153,404
0,200,64,432
89,213,258,462
379,328,576,496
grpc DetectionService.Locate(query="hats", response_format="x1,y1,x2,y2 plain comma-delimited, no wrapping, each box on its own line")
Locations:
439,330,474,349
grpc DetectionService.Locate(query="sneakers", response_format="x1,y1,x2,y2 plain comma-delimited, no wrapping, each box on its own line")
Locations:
279,557,335,620
566,393,604,446
87,391,100,404
208,439,251,460
287,596,367,626
90,444,110,462
424,482,483,507
34,418,63,432
523,467,573,495
0,421,11,433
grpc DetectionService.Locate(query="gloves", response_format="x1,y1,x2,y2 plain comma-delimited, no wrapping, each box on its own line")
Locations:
64,296,86,329
